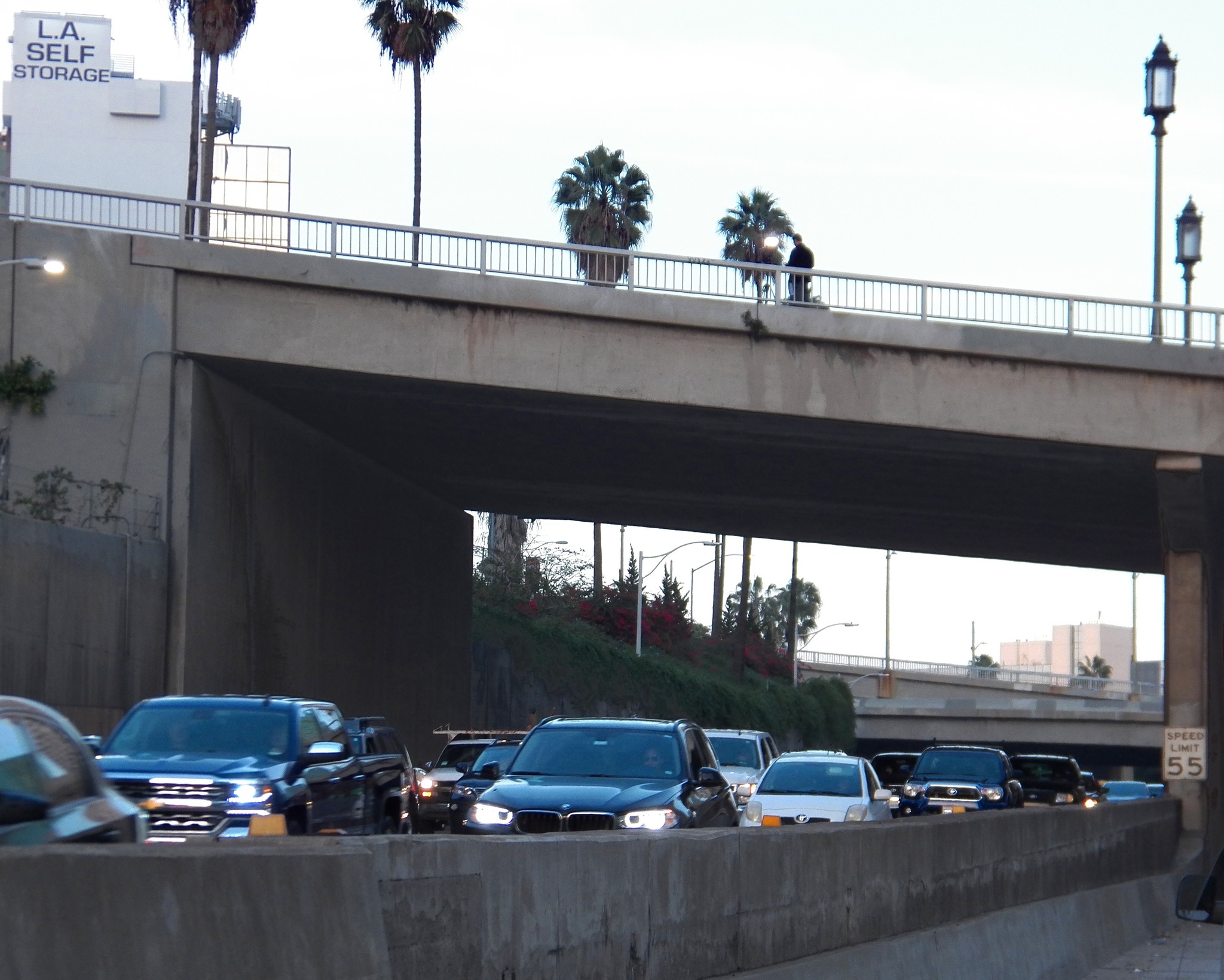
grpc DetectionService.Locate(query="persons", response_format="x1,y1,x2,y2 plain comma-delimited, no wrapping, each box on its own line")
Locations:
636,748,676,779
784,233,814,306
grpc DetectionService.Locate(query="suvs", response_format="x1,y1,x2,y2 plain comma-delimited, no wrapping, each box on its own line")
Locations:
687,728,775,818
89,694,382,834
472,715,740,829
324,715,424,833
893,742,1032,816
447,737,524,838
426,731,499,834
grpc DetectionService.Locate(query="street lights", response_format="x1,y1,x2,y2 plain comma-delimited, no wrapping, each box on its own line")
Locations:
631,535,893,690
754,234,780,309
1176,194,1201,346
1142,33,1177,345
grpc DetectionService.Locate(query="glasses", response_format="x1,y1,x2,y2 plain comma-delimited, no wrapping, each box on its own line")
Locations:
643,755,661,762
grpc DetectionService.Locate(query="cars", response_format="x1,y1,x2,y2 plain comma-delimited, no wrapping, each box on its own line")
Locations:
1103,776,1166,802
286,699,1106,784
1,689,149,849
737,750,897,828
1006,745,1089,810
